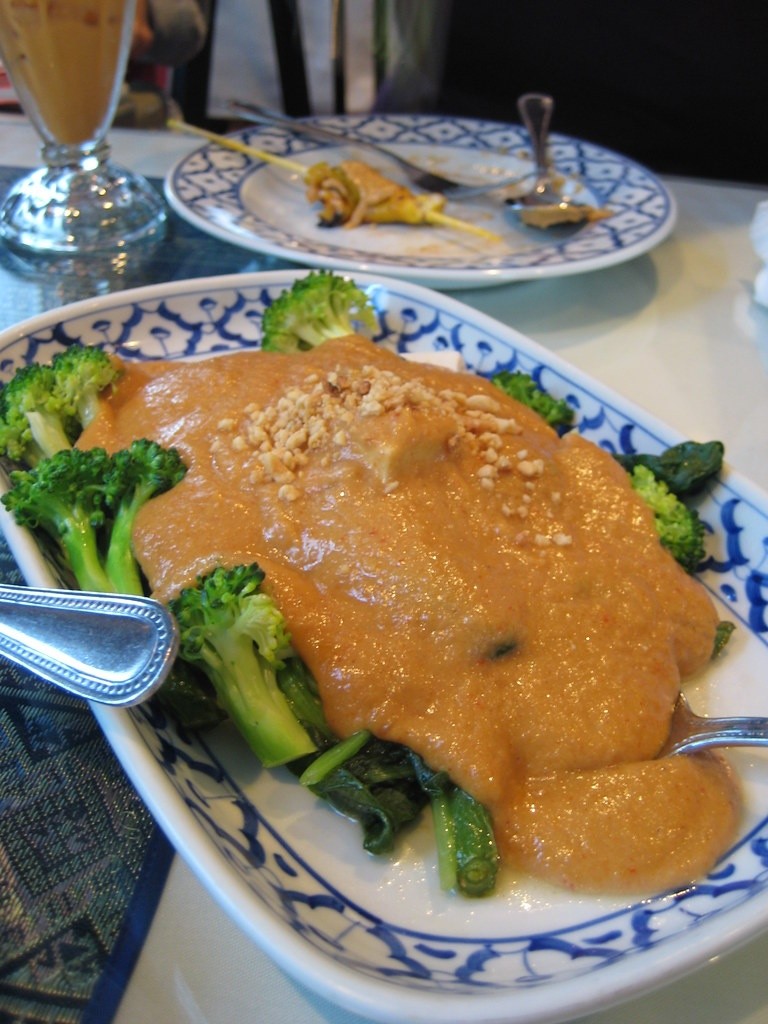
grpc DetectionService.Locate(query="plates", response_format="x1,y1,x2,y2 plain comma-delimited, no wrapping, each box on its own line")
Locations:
165,113,678,290
0,272,764,1023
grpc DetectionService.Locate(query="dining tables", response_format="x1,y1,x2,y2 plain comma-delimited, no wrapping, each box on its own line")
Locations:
1,109,766,1023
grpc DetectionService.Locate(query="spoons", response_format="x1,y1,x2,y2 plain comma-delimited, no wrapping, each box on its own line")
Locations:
506,94,613,231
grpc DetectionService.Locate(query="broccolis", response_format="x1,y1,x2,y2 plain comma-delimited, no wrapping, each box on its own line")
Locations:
0,271,706,766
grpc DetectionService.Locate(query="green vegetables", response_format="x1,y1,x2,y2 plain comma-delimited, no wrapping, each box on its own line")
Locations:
136,437,724,889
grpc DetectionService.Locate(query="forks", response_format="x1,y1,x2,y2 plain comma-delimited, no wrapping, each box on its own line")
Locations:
224,99,545,200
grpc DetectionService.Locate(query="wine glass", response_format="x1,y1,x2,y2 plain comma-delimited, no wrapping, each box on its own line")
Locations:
2,0,167,254
